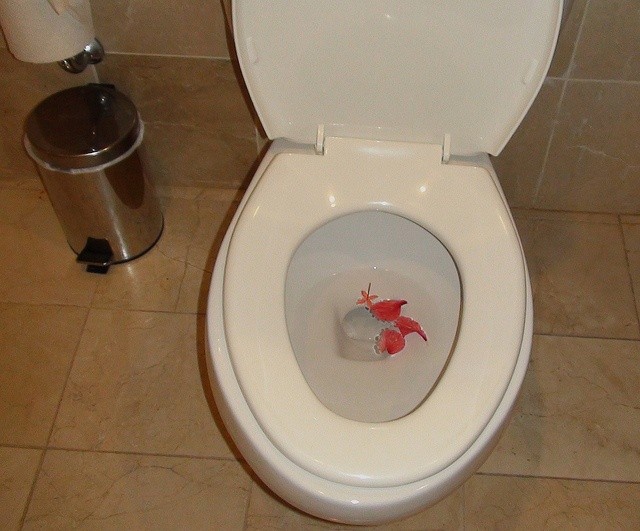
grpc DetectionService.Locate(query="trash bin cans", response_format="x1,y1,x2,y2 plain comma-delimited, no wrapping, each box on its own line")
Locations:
22,82,165,268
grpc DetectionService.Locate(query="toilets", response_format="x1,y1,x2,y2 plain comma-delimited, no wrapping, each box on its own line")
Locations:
204,0,563,526
